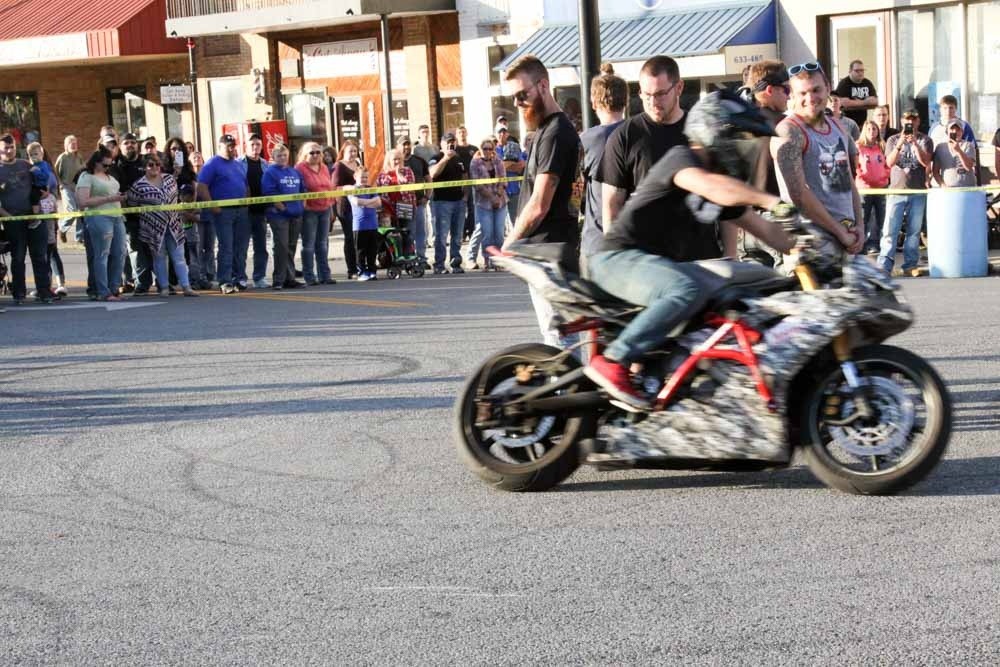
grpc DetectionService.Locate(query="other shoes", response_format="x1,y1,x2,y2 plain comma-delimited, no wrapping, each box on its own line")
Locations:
869,246,878,254
904,268,921,277
0,226,498,307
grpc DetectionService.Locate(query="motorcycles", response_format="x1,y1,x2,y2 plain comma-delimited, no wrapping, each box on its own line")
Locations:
451,204,956,498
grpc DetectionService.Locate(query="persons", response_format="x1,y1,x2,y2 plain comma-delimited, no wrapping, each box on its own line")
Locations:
873,105,899,141
503,56,583,361
853,120,890,235
55,135,85,243
603,55,738,259
769,61,865,254
825,92,861,141
0,133,68,306
76,124,381,302
584,90,799,406
746,61,791,196
580,73,629,256
928,95,977,187
836,60,879,128
877,109,933,277
376,115,536,275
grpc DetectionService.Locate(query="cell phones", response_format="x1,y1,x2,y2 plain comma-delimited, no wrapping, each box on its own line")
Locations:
175,152,183,166
450,145,454,150
950,133,956,143
904,123,913,134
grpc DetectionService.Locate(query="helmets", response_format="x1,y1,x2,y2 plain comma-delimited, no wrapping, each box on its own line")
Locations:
683,91,781,183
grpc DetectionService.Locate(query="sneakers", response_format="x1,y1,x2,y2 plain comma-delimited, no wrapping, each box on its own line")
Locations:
583,355,650,409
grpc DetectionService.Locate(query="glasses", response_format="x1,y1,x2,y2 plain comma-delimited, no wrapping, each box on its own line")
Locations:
482,147,494,151
761,83,790,97
903,112,919,118
307,150,322,156
639,81,679,102
511,78,541,102
851,68,865,74
99,146,183,169
787,62,825,79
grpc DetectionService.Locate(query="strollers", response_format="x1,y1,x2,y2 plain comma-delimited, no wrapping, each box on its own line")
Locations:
355,198,427,280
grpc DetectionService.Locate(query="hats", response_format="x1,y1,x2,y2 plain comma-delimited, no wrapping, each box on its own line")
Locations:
218,134,237,148
496,125,509,134
101,136,117,147
144,135,156,148
946,118,963,129
441,132,456,144
397,136,410,147
119,133,137,144
496,115,508,122
902,108,920,120
